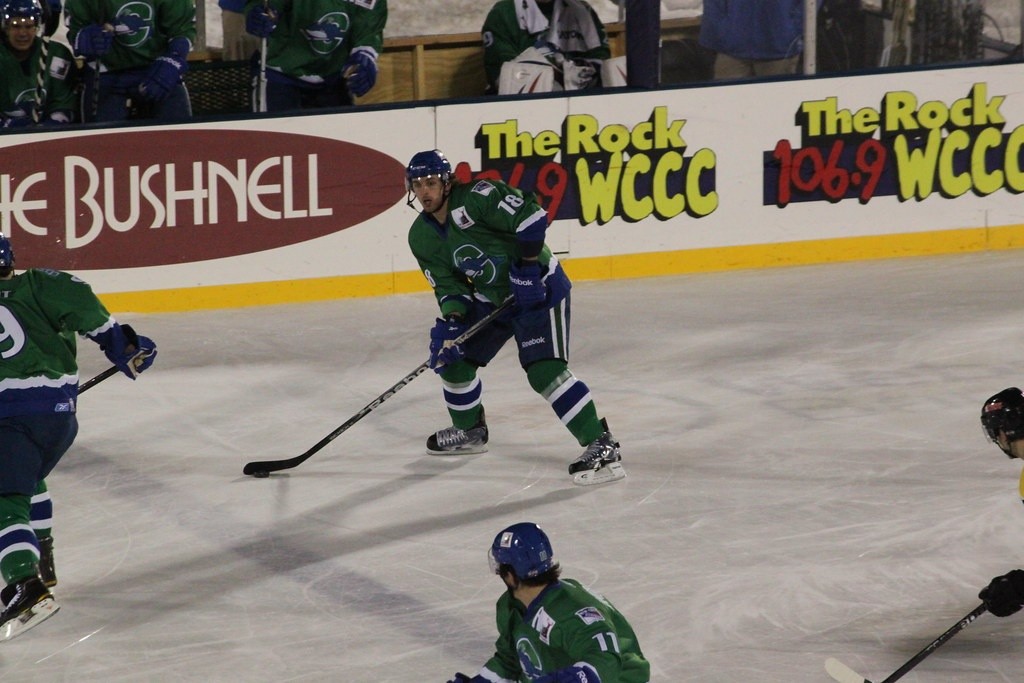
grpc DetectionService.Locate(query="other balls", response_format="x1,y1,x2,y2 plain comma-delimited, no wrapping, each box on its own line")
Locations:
253,471,270,478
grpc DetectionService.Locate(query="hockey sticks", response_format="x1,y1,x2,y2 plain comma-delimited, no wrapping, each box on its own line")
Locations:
90,0,107,122
260,0,268,113
77,365,120,396
823,603,987,683
243,296,516,476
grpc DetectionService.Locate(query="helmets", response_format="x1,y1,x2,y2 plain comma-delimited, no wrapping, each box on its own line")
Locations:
489,520,553,580
981,387,1024,458
0,0,44,34
407,149,451,193
0,232,16,268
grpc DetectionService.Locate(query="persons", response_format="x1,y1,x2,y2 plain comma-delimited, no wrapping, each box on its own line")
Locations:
448,523,650,683
696,0,805,81
978,387,1024,616
0,232,159,626
0,0,197,136
796,0,882,78
481,0,611,95
218,0,388,112
406,148,622,476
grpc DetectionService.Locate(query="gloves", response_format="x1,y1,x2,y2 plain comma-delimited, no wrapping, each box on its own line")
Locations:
536,661,600,683
101,324,156,380
342,46,377,98
980,569,1024,617
509,258,547,309
36,110,69,127
73,24,112,58
447,672,491,683
143,52,183,103
429,318,467,374
245,5,276,37
0,105,36,128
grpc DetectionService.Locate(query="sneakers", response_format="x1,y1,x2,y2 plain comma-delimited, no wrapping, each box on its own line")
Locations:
427,403,488,455
569,417,625,486
35,535,58,595
0,574,59,644
